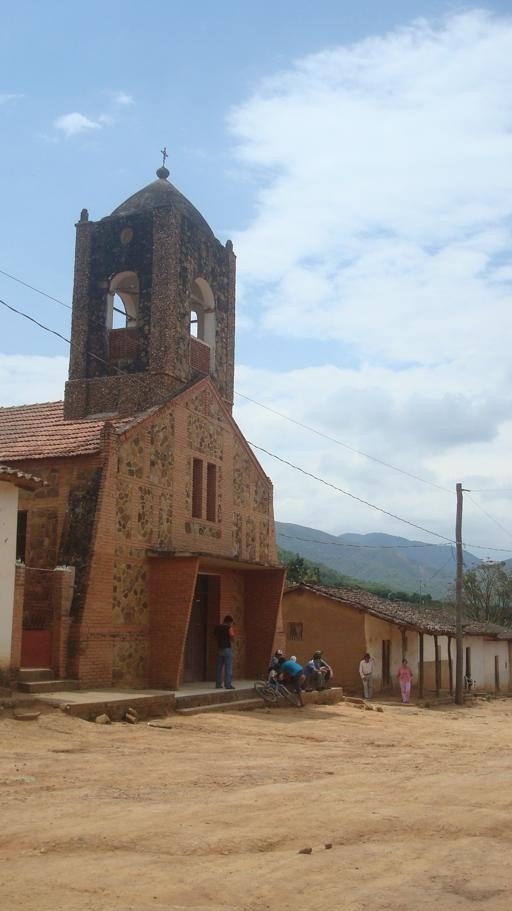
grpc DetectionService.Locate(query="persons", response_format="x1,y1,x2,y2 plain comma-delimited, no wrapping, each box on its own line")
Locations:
276,658,308,708
302,650,333,691
396,659,417,702
268,651,285,684
213,616,235,689
466,673,473,691
359,654,376,699
282,655,302,683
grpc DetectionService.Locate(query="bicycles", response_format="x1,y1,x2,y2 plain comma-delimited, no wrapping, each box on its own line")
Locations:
254,675,300,708
